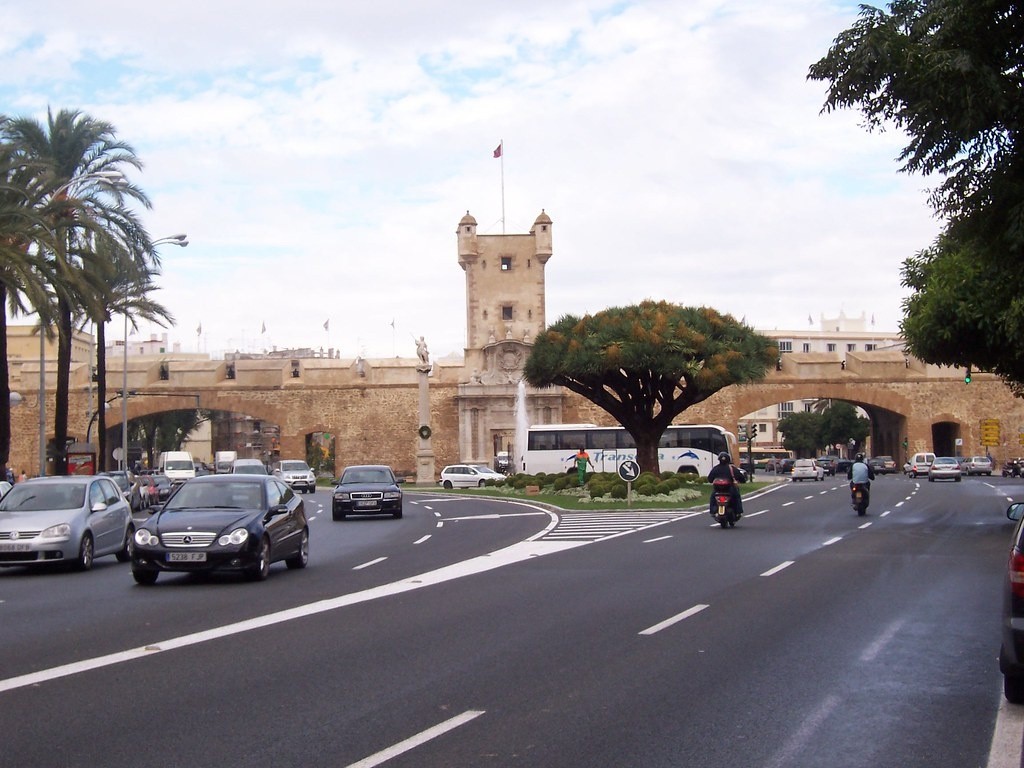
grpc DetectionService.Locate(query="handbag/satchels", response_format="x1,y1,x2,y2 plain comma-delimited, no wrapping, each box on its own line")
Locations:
733,480,740,490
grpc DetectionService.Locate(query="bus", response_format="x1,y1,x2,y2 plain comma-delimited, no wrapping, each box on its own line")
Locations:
523,424,737,482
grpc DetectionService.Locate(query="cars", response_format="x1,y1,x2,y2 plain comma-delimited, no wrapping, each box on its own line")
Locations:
330,465,406,520
0,471,175,572
999,502,1024,706
194,462,214,476
131,475,310,586
736,456,993,483
439,464,510,489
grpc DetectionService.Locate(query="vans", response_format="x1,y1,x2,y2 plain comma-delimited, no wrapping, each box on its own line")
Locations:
229,459,271,498
494,451,508,473
156,451,196,481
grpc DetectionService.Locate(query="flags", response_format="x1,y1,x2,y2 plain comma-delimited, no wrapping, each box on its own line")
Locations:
493,144,502,158
809,316,813,326
195,323,202,336
739,316,746,326
390,319,394,328
871,315,877,328
262,322,267,334
323,319,329,331
128,325,136,337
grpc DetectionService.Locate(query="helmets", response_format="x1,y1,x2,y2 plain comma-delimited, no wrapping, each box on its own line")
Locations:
855,454,864,461
718,452,731,463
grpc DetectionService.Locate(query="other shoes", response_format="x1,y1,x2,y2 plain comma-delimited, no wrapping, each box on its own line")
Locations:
736,514,742,518
710,513,715,517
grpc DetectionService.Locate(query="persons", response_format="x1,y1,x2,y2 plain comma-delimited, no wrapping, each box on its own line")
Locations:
986,453,992,462
6,468,15,486
847,453,875,509
707,452,746,520
20,470,27,481
574,448,595,486
415,336,429,364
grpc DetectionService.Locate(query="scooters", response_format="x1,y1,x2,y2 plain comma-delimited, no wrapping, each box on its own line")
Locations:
1002,457,1024,479
713,476,741,528
851,487,871,515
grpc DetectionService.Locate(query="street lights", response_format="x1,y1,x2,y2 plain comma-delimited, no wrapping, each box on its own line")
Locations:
122,232,190,471
38,171,128,475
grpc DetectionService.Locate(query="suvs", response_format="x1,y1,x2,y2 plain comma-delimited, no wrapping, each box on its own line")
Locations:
269,460,317,493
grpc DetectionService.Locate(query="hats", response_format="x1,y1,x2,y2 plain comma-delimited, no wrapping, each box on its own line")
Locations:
9,468,13,470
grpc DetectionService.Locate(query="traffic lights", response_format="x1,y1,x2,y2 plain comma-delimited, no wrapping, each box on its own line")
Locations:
904,437,908,447
965,363,972,383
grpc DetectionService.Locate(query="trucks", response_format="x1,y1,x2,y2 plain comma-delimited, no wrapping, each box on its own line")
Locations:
214,451,237,475
